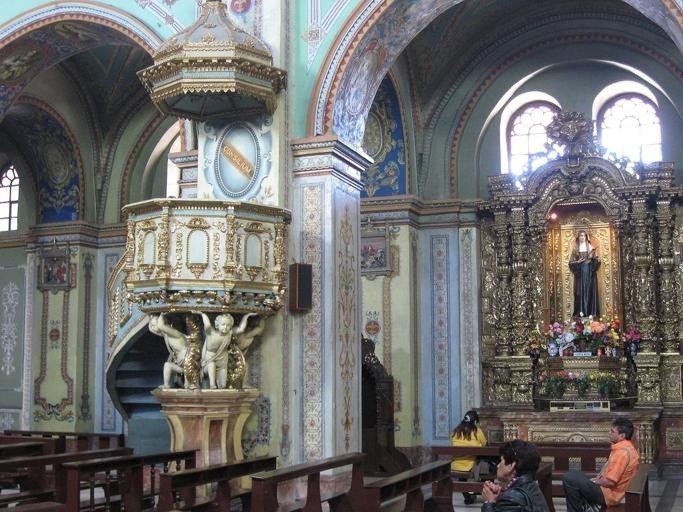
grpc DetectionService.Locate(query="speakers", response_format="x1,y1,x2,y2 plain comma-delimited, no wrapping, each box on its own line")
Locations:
289,263,313,315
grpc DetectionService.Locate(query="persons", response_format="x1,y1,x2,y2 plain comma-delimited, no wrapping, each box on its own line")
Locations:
146,311,188,391
480,438,552,512
567,230,602,319
237,314,271,390
190,310,260,391
450,410,495,506
561,417,641,512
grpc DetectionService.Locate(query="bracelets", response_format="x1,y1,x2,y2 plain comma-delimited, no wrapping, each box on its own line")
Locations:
592,480,595,484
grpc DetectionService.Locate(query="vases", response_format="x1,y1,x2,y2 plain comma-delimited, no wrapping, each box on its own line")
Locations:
547,341,638,357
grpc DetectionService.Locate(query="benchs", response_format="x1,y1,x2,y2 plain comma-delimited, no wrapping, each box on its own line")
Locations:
0,435,652,512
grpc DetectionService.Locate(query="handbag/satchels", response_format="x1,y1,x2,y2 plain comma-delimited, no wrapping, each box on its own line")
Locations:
581,477,603,512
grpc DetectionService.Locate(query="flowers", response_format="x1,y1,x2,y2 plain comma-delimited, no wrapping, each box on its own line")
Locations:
545,313,642,347
